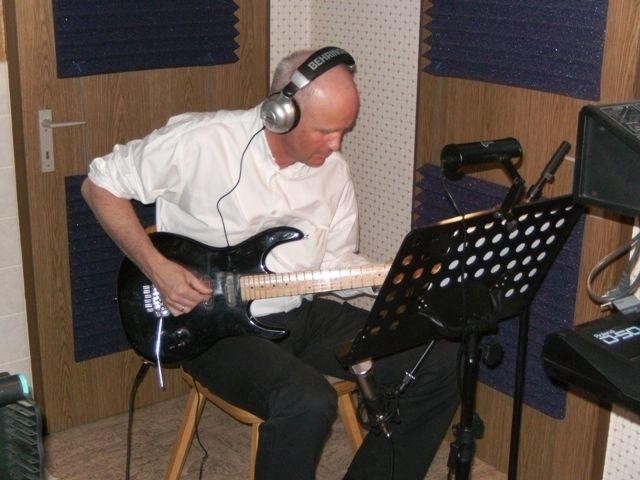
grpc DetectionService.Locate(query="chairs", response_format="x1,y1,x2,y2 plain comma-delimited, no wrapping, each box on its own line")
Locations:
145,222,368,479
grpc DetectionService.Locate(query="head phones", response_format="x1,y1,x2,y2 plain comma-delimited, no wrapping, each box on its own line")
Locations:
260,46,356,135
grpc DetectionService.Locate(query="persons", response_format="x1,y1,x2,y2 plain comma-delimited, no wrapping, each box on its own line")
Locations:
80,42,471,480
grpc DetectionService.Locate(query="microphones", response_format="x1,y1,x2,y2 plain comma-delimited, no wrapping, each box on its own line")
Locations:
352,358,393,438
526,138,572,201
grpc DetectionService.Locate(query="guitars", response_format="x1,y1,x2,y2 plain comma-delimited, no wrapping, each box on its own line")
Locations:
117,226,394,368
